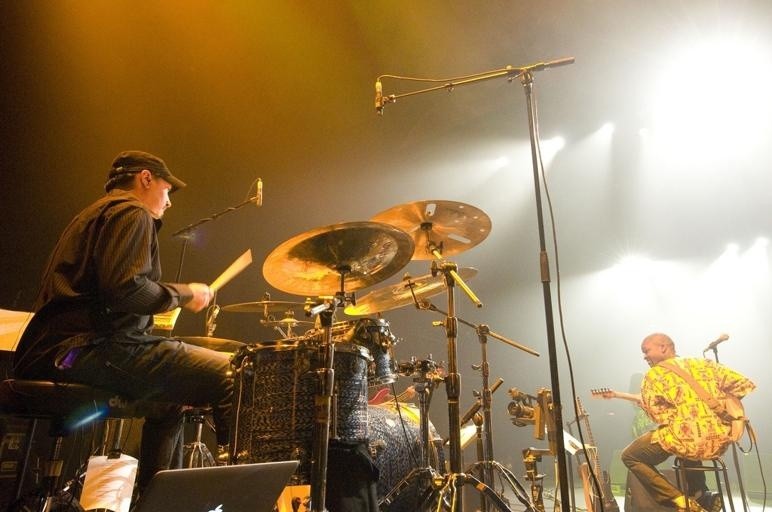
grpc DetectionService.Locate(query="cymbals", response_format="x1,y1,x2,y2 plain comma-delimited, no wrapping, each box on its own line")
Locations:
260,318,315,327
372,201,491,261
263,220,416,297
219,301,320,315
343,267,480,315
162,336,251,352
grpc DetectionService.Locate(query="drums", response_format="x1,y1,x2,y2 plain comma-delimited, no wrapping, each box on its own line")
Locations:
305,319,398,388
254,339,373,478
230,343,257,464
313,402,447,511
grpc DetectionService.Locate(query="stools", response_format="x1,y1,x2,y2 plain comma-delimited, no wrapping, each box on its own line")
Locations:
0,380,147,512
672,456,736,512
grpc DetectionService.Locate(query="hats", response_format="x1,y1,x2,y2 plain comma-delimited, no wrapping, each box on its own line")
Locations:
111,151,186,192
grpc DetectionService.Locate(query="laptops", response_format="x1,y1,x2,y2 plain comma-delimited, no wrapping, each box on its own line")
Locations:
134,460,300,512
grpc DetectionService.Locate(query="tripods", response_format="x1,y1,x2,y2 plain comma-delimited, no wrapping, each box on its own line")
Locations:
378,274,535,512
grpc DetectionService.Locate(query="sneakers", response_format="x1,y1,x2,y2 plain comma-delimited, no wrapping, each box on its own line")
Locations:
676,501,707,512
697,491,722,512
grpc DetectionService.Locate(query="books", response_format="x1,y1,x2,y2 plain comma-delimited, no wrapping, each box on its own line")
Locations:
444,423,484,448
544,425,584,456
0,309,36,352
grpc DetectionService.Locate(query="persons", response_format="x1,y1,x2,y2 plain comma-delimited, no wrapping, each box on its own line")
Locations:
17,151,237,489
620,332,756,512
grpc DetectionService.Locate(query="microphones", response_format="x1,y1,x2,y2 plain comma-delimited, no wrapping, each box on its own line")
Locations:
309,296,343,317
375,81,384,115
709,333,729,348
256,178,264,207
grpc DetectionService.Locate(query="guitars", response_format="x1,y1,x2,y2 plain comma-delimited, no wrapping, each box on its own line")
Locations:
576,398,619,512
590,389,745,445
